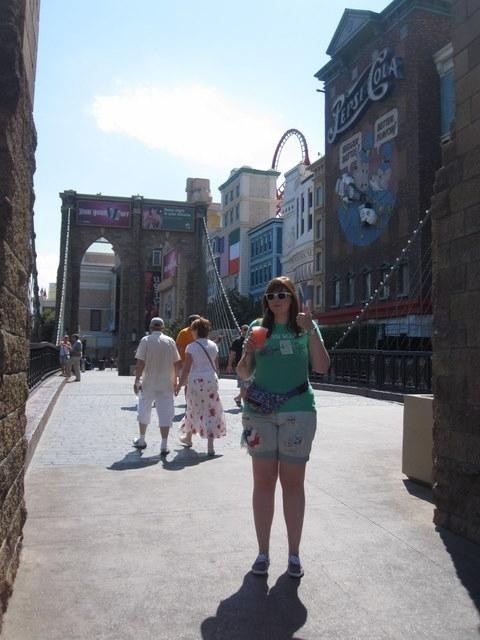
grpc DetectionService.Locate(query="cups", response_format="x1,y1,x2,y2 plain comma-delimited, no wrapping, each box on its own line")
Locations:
252,326,268,350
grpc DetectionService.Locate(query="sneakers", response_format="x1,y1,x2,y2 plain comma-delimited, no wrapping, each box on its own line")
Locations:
179,436,192,446
133,437,146,448
252,553,270,575
287,554,303,577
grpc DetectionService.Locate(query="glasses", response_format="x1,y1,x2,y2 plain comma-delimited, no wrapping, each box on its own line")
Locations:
244,328,247,331
265,291,293,301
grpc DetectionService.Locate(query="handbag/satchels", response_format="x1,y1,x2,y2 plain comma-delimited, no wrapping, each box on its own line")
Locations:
244,383,288,416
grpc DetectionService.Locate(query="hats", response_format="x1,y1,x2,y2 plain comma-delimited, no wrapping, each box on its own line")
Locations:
150,317,164,329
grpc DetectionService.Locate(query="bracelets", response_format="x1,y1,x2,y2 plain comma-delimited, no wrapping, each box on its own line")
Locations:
305,324,319,335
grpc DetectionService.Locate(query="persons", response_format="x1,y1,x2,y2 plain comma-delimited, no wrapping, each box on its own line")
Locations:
131,311,227,456
67,333,84,381
57,334,73,377
226,323,250,407
143,206,163,228
81,355,87,372
235,274,331,577
